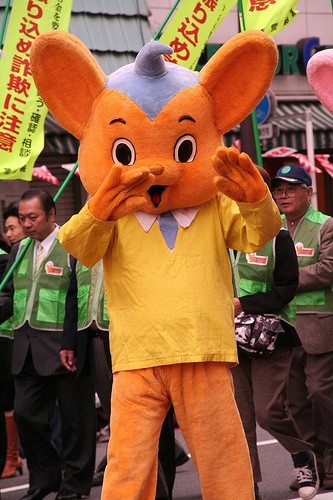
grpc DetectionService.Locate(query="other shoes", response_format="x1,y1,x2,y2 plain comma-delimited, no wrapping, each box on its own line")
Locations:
55,489,81,500
173,440,188,466
20,483,60,500
92,455,108,486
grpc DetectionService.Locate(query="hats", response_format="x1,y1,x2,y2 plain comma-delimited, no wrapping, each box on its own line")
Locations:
271,164,312,187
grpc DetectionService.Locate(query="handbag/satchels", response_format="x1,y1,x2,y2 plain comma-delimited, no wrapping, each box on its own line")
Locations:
235,312,284,354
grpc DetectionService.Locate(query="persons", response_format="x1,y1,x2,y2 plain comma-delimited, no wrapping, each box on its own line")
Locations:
272,165,333,490
0,196,24,479
0,189,95,500
59,252,177,500
230,228,319,500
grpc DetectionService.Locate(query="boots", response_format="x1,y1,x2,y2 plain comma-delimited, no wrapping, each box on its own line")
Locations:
1,416,24,478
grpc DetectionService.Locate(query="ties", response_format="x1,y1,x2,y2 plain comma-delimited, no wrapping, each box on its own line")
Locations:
36,244,43,272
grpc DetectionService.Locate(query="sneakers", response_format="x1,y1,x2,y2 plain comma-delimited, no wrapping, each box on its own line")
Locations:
291,451,320,500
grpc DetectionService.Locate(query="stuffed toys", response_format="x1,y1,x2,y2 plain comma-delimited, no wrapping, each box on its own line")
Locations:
29,31,278,500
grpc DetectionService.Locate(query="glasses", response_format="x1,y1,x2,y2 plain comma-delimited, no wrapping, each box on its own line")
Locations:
271,188,307,198
